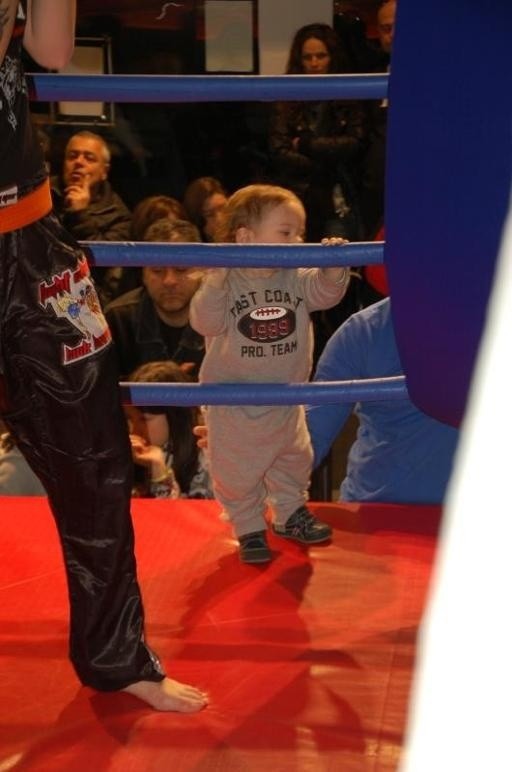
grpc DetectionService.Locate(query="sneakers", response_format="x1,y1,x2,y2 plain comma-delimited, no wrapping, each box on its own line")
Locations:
273,506,333,543
238,530,271,564
